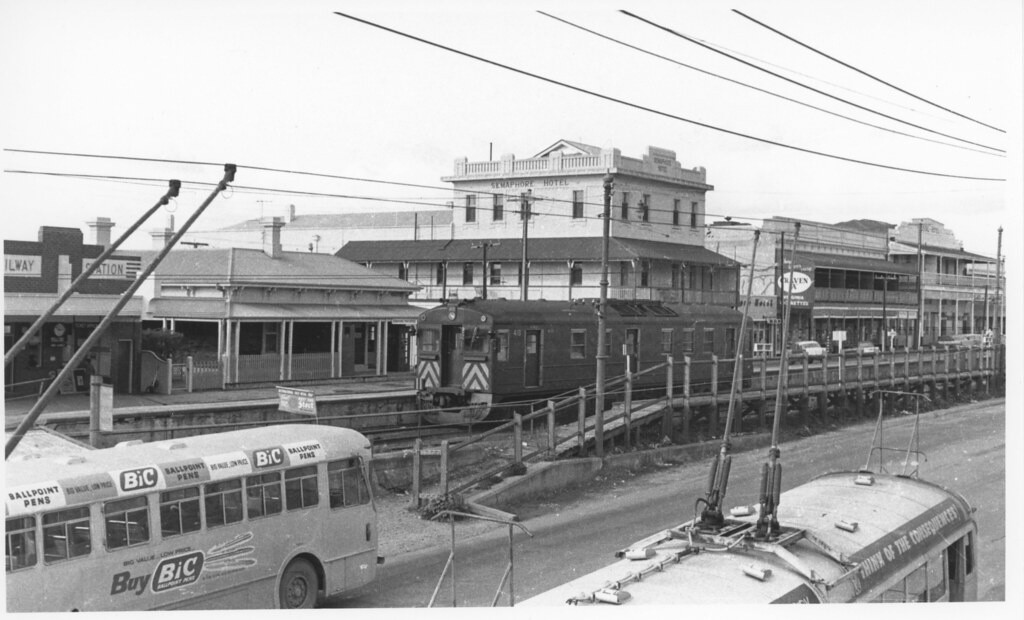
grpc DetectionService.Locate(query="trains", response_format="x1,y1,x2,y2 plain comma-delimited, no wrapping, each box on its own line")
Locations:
402,299,757,426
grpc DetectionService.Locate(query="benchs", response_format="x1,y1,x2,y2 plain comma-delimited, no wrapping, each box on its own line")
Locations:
6,489,317,569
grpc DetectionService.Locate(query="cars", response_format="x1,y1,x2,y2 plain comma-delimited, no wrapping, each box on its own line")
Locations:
787,341,828,365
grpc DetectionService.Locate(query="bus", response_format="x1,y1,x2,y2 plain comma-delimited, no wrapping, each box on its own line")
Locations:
5,424,380,612
509,469,981,604
